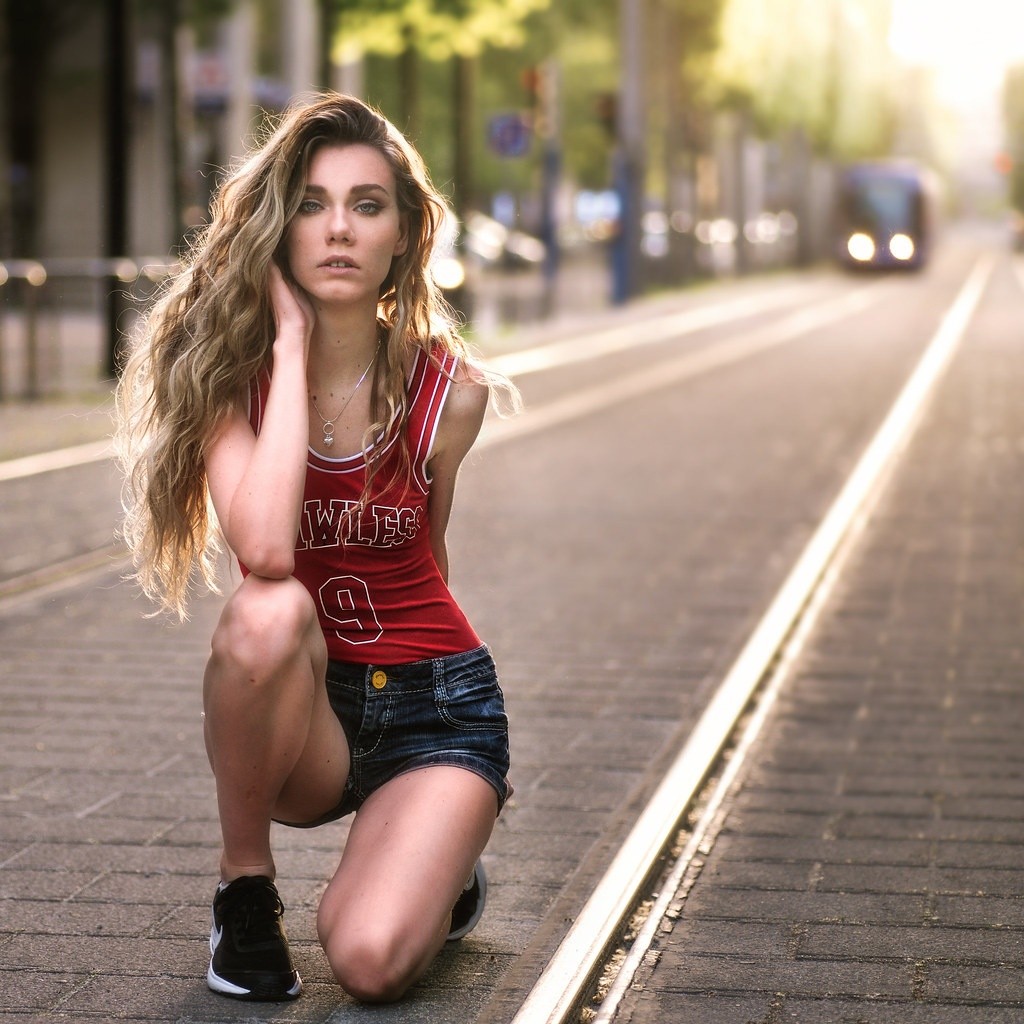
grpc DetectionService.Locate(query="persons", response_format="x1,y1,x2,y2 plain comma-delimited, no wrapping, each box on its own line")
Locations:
113,96,523,1001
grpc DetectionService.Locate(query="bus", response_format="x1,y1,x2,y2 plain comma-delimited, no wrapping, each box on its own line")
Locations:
835,166,940,270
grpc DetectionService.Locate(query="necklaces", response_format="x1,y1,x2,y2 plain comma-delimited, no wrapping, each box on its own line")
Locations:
310,339,382,448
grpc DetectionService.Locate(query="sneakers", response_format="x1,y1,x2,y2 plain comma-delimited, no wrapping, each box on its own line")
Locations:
447,858,488,940
207,876,303,1001
